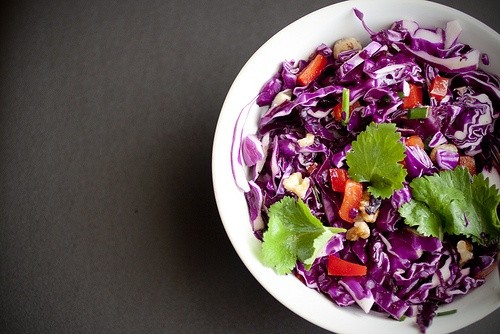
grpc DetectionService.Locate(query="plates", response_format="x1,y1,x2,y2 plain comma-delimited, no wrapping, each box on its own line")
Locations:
211,0,499,334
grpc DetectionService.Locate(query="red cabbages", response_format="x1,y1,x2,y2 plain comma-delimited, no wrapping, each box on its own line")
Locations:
238,7,500,334
